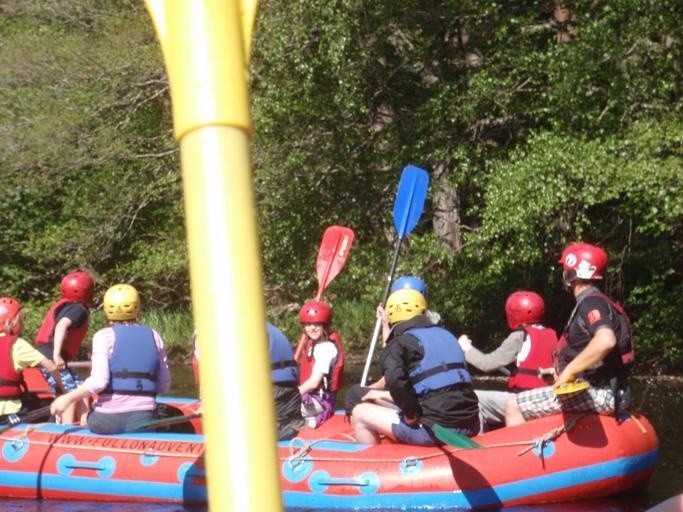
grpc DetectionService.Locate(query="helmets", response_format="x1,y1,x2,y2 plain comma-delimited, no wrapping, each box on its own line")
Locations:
557,243,610,282
298,301,333,329
0,295,22,335
384,275,429,328
60,271,95,305
503,290,546,330
102,283,141,321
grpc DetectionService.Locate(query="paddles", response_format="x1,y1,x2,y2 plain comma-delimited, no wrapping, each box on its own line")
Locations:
359,166,428,391
373,398,484,449
293,225,353,360
127,413,201,432
553,381,590,395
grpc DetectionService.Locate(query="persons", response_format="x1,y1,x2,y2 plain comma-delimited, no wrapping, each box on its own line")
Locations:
266,319,305,440
295,302,346,428
504,243,636,430
457,289,558,435
1,296,64,425
346,275,428,420
50,284,172,434
34,271,94,397
351,287,481,444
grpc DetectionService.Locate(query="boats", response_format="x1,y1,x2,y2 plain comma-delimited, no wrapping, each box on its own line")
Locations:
0,394,660,510
